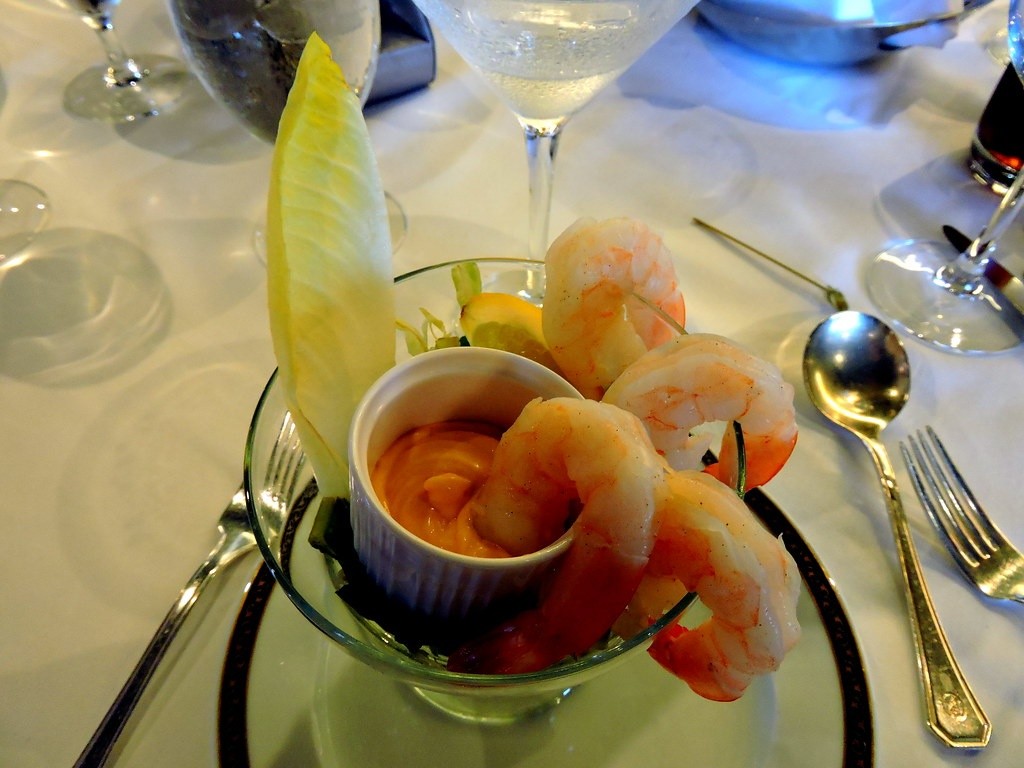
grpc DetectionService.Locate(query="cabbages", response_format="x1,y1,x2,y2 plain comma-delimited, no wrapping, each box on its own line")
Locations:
266,33,396,496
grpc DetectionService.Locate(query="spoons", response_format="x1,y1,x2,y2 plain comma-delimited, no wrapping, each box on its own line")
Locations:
802,309,990,749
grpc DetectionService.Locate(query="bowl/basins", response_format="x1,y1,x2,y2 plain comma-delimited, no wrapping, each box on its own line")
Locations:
352,349,593,615
694,0,993,68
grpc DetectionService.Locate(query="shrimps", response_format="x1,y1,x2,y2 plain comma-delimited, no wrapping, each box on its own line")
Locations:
423,214,801,704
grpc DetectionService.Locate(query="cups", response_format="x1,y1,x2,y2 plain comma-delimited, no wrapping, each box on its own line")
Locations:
969,62,1024,203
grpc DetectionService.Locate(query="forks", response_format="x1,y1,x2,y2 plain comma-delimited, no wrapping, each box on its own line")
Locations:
68,409,305,768
899,426,1024,603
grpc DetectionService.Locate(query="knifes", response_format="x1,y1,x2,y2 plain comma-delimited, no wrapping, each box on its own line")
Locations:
942,224,1024,317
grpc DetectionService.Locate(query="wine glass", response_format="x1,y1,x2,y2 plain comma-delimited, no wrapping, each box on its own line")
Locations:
870,0,1024,353
8,0,188,121
242,259,780,767
164,0,407,269
412,0,702,332
